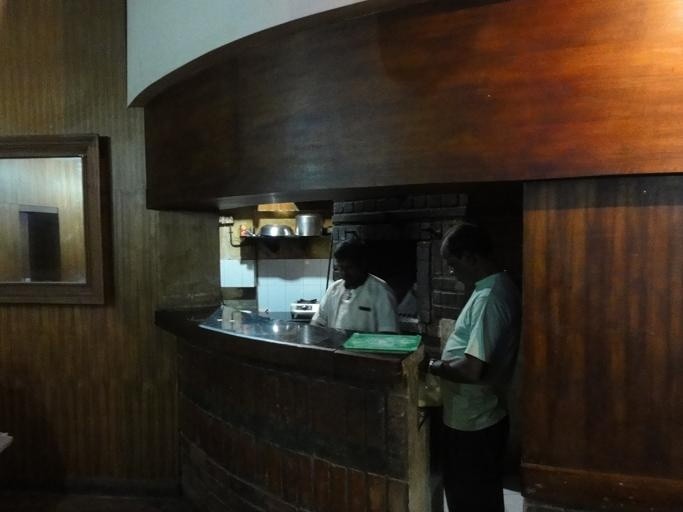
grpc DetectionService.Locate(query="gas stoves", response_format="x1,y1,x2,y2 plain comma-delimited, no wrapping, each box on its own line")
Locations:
292,299,323,318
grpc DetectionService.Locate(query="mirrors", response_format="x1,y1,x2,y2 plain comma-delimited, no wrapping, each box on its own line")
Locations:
1,136,112,307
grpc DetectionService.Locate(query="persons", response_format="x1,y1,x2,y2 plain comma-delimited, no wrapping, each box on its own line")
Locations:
425,224,522,512
310,238,402,334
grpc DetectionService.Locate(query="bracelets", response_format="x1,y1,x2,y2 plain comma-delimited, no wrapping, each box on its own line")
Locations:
427,358,437,372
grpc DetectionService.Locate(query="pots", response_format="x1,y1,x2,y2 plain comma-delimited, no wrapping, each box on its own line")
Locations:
294,215,325,238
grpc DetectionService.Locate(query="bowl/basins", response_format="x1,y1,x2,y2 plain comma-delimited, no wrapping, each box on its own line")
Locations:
260,223,293,237
